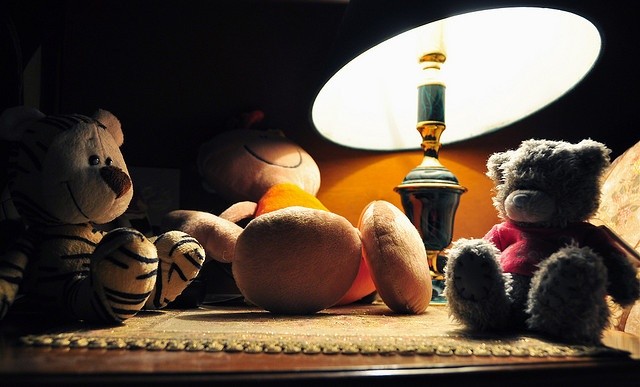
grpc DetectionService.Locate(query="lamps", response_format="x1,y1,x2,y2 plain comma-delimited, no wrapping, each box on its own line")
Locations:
311,6,606,305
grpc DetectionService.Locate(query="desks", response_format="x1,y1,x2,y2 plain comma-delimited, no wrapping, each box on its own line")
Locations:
0,295,640,386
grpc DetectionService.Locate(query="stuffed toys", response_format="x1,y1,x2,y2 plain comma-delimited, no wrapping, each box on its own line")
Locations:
1,98,205,326
442,138,636,346
158,110,433,316
